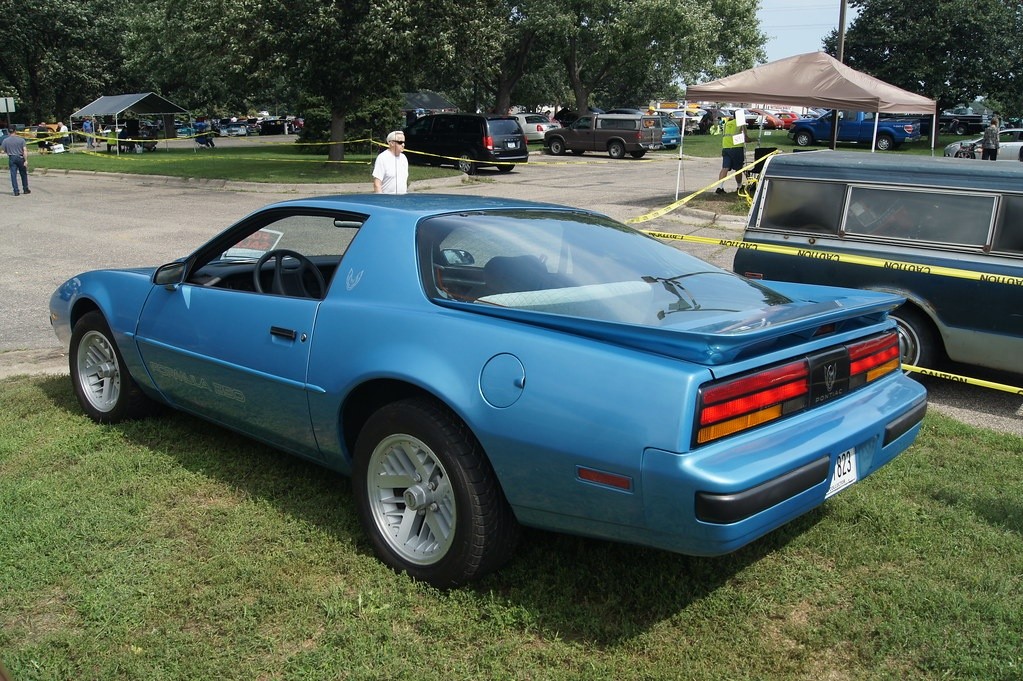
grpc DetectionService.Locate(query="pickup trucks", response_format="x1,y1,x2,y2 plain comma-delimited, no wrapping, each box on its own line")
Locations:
877,111,988,135
787,106,922,151
728,109,759,128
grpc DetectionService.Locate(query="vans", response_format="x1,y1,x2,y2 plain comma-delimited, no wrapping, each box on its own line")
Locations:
730,149,1023,393
260,119,291,135
648,109,691,132
401,113,529,174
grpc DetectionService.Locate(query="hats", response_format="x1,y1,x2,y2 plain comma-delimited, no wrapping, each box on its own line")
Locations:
8,125,16,133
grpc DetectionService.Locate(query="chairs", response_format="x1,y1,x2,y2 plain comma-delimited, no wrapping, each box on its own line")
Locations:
746,147,778,188
482,254,550,297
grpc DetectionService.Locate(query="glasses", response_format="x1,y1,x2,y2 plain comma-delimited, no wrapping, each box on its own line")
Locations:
389,140,406,145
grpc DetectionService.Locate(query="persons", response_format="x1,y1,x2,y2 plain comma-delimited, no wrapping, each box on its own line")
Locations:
37,122,50,155
91,118,102,147
0,125,31,195
372,131,409,194
58,122,69,154
714,109,757,194
645,106,656,127
981,116,1000,161
106,125,158,152
83,117,94,149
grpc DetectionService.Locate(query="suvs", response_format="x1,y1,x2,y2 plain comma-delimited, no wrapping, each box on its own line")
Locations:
685,109,708,129
544,112,663,160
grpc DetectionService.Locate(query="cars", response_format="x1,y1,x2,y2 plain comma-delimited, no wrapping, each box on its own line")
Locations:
512,114,560,144
944,128,1023,160
704,109,733,122
749,109,784,129
47,191,930,594
654,116,681,150
772,112,800,130
227,122,248,137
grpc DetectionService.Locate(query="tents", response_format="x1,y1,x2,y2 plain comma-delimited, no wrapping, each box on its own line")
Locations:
674,51,937,203
70,92,196,156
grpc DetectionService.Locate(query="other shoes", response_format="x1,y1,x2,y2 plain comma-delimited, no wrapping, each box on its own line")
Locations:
716,188,726,195
24,190,31,194
15,193,20,196
737,185,746,191
